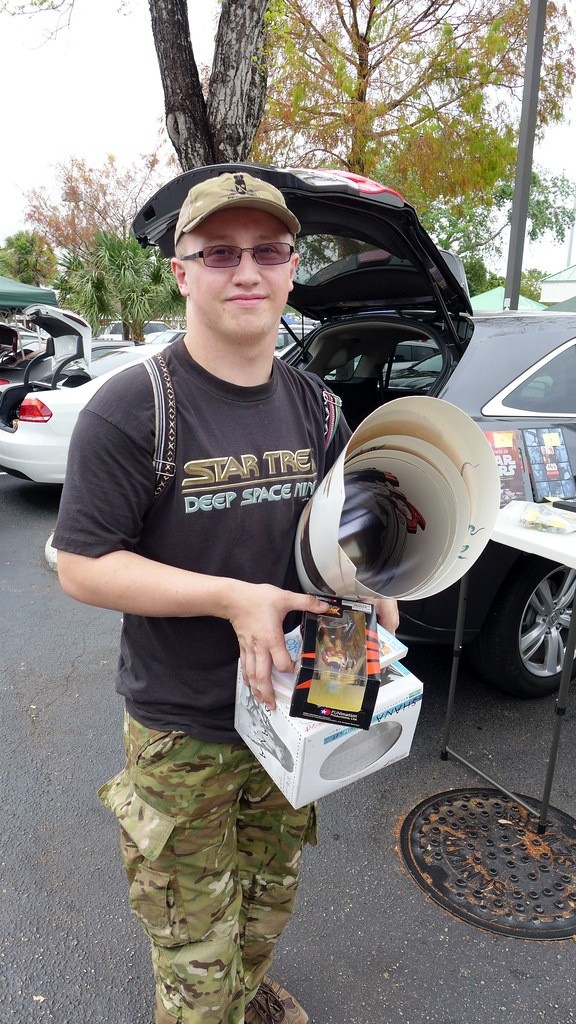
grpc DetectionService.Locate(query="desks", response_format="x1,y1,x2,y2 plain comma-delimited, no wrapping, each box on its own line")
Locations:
441,500,576,834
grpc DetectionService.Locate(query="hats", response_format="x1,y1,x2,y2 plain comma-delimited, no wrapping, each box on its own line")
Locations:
174,172,301,257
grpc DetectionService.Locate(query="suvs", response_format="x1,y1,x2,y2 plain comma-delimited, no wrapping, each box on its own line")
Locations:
131,161,576,704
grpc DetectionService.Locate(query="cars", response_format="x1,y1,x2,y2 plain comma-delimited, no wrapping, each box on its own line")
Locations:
274,313,443,393
0,300,187,485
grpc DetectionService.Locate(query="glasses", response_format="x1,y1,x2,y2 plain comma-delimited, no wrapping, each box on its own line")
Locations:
181,242,295,268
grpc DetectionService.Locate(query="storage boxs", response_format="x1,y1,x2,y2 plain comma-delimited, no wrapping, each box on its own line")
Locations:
234,624,423,810
288,595,381,731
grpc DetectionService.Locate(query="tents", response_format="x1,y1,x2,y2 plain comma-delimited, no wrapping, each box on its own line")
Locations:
0,276,57,308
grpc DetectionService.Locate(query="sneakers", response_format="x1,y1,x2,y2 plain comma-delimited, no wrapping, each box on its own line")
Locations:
245,976,310,1024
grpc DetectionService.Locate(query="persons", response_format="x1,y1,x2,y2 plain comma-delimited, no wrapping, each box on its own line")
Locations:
51,170,401,1024
